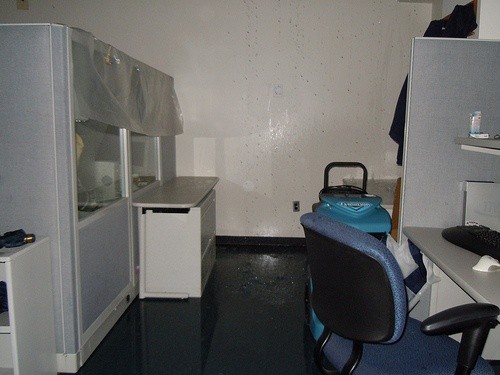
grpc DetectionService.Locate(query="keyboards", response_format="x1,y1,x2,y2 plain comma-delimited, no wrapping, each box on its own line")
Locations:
442,224,500,262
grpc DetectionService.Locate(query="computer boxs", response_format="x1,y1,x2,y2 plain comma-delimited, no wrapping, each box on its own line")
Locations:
461,178,499,231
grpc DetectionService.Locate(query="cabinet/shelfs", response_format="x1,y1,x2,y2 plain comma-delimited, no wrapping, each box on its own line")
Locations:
132,176,220,299
0,235,57,375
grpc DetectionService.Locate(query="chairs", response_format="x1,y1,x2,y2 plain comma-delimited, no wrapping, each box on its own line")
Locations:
300,212,500,375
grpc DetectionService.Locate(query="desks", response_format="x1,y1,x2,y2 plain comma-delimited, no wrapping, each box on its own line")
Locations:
403,227,500,323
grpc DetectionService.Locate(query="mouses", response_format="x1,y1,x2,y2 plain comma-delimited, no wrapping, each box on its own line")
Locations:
473,255,500,272
